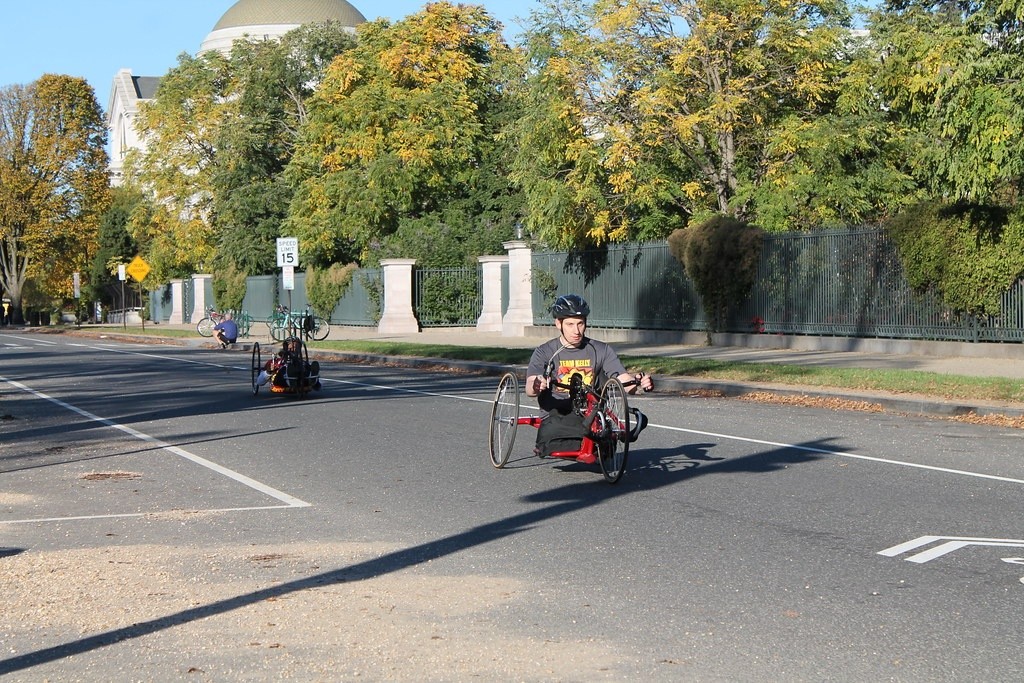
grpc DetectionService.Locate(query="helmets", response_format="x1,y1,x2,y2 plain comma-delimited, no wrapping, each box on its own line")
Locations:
283,336,302,351
552,294,590,319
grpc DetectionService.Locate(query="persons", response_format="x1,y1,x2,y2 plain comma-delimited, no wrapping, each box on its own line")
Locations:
524,295,652,454
256,336,320,386
212,313,237,349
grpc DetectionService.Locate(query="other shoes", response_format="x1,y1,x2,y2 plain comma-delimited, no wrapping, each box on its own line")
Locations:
215,343,225,349
228,344,233,349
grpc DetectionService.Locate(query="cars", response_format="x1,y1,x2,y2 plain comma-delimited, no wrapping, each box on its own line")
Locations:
58,312,77,324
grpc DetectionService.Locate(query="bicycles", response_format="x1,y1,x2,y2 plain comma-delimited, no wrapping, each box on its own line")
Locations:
196,305,249,338
233,315,254,328
267,303,331,342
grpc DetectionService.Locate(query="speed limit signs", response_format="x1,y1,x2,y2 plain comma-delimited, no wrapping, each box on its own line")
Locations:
276,237,298,267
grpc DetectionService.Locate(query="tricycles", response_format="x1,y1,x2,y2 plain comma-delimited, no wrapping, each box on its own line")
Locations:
489,371,648,485
252,336,321,400
